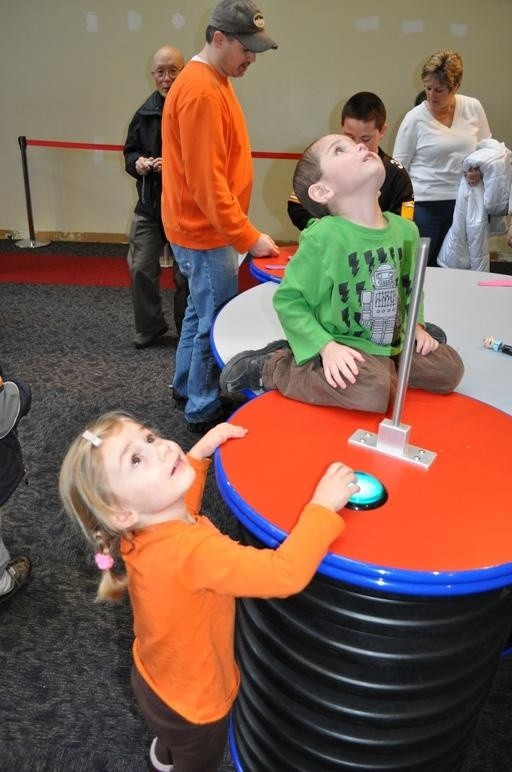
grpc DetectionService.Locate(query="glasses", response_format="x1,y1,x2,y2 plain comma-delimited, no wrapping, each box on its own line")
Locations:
150,67,184,80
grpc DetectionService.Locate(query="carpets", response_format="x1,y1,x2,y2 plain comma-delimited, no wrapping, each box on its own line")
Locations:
1,278,512,771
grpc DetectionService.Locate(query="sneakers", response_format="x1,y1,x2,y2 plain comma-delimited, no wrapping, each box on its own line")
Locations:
171,393,236,434
149,736,174,772
426,322,446,343
133,326,169,347
0,554,31,603
219,339,293,394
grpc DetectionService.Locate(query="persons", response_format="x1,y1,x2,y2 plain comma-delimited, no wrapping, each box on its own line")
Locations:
286,91,416,233
156,1,283,435
389,47,494,270
121,44,191,349
57,409,364,772
218,132,467,417
0,376,34,605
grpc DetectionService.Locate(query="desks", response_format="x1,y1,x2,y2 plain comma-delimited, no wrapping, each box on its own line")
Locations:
213,382,512,771
206,266,511,424
247,237,301,284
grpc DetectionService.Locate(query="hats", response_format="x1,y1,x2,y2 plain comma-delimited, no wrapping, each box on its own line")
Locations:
208,0,277,52
